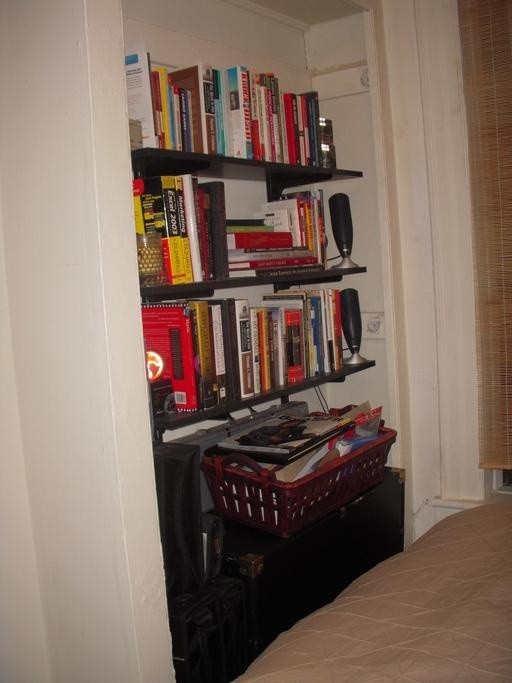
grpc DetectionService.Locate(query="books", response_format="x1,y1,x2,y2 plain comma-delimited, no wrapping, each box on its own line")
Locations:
132,174,328,287
141,288,344,415
124,51,337,170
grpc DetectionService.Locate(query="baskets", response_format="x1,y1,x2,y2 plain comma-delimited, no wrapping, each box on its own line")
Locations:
202,414,398,538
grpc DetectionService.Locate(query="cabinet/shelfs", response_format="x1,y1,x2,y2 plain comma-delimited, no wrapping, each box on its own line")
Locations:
131,147,376,429
221,470,405,683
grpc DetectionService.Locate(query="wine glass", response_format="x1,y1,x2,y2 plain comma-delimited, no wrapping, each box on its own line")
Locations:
136,232,167,288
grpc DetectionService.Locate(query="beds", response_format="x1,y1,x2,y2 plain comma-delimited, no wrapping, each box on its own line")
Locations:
229,499,512,683
